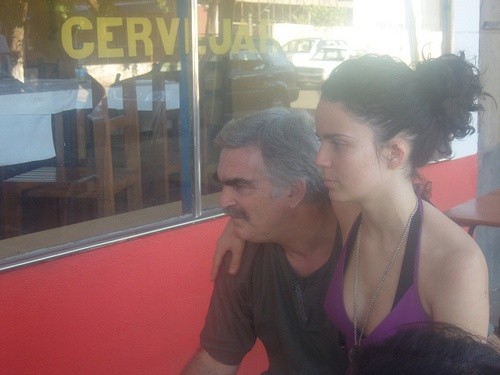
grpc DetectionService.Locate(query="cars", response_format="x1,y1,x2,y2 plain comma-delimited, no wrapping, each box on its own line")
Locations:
279,37,350,69
105,34,301,134
295,45,374,89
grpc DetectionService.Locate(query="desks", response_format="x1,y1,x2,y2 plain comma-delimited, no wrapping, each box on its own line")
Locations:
443,189,500,241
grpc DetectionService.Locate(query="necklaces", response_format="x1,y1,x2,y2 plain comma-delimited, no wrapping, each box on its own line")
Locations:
349,198,418,362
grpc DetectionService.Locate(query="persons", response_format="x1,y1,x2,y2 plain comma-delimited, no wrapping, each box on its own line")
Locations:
180,108,349,375
353,321,500,375
0,13,105,123
212,51,489,375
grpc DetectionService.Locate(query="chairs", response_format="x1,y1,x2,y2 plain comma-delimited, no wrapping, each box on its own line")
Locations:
3,75,141,238
139,62,182,207
